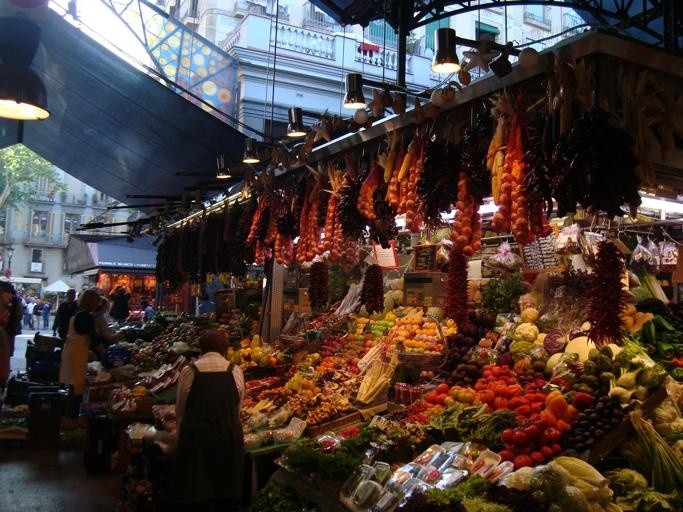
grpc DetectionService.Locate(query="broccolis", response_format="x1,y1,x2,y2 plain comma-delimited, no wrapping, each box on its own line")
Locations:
510,306,548,361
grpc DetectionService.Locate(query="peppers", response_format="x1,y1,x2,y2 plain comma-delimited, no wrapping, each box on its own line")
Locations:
585,240,626,349
641,315,683,382
156,152,397,293
442,247,478,335
358,264,386,314
416,107,641,226
308,262,330,312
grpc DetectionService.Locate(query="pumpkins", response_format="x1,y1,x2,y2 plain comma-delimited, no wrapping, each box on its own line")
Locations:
417,379,593,432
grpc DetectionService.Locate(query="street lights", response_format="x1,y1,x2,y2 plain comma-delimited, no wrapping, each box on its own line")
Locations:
6,244,13,272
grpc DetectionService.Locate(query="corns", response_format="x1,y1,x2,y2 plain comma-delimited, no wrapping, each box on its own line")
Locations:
548,455,623,512
651,406,683,435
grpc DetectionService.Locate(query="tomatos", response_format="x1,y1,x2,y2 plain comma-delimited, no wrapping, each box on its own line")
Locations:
498,415,562,469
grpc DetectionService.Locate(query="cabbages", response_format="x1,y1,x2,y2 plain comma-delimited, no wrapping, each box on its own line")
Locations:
528,313,568,365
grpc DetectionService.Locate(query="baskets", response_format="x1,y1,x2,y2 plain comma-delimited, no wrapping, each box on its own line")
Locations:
396,313,448,371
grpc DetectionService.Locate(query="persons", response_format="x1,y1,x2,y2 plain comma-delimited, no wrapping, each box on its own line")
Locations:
174,331,245,511
109,285,131,318
198,296,215,314
52,290,75,341
10,285,22,358
92,294,108,329
139,300,154,321
58,290,122,423
16,290,50,331
0,281,12,396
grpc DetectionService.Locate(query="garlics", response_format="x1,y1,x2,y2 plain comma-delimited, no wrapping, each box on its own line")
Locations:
245,153,553,268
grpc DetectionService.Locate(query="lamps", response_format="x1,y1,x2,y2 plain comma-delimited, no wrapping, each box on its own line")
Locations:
432,26,521,74
343,73,416,108
212,136,280,178
0,1,50,128
286,105,334,138
